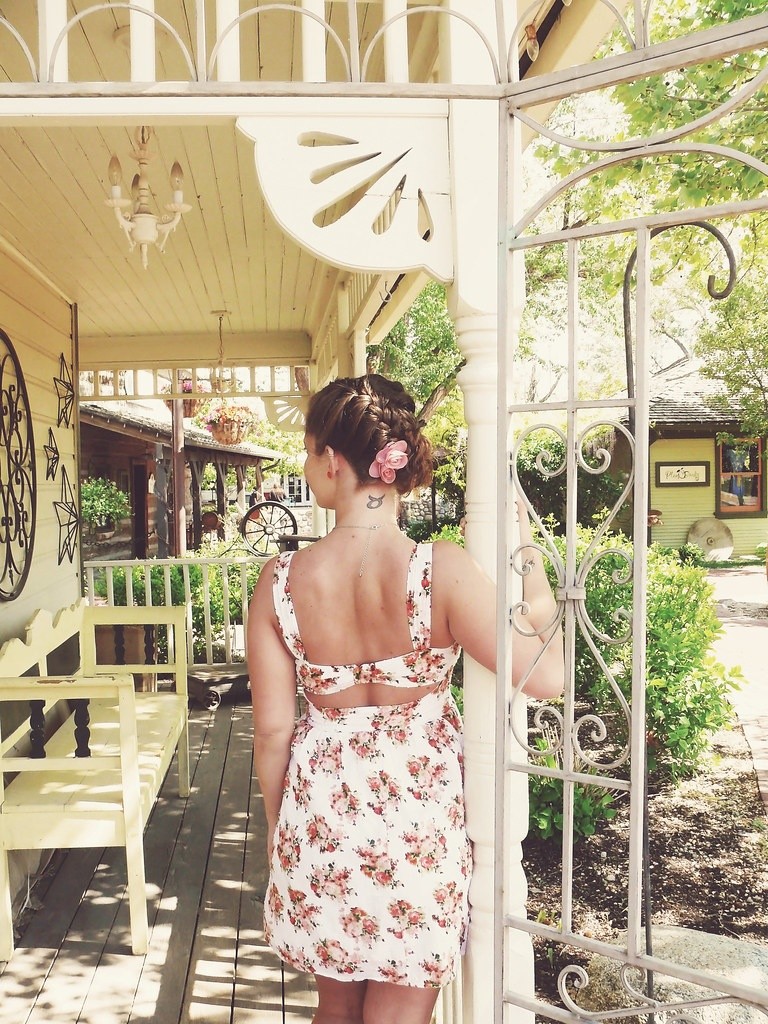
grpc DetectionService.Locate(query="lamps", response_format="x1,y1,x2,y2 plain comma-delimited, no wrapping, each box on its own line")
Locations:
138,442,153,461
207,310,238,400
105,125,192,270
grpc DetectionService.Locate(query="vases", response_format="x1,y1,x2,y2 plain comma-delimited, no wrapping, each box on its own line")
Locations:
163,399,206,417
94,627,159,694
211,419,246,444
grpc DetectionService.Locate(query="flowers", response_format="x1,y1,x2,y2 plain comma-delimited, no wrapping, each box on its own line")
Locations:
191,402,260,431
161,379,204,395
368,440,409,485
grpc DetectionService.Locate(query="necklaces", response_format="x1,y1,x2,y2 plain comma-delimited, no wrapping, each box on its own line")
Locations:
333,524,392,577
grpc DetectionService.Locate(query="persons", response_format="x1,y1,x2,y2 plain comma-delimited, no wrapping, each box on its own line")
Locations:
249,486,258,507
245,373,564,1024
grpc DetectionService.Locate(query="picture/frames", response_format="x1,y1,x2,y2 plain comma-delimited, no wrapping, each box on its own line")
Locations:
655,461,710,487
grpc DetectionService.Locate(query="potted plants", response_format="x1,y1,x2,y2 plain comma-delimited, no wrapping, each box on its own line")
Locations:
80,478,136,542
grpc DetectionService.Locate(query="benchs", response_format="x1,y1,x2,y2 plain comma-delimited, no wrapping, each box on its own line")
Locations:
0,595,191,963
